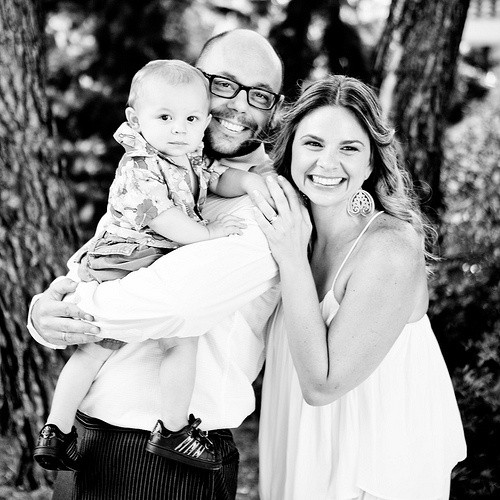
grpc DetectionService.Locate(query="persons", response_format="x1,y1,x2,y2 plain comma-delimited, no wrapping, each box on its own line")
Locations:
251,76,466,499
26,28,285,500
33,59,277,472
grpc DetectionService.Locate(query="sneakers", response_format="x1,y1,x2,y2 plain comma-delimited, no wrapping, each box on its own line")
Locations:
146,414,223,471
33,424,83,473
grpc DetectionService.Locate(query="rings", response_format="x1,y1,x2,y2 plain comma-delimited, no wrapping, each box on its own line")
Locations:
63,332,67,342
269,215,278,224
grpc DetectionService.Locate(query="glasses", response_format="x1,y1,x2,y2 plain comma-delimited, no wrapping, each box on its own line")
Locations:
196,68,280,111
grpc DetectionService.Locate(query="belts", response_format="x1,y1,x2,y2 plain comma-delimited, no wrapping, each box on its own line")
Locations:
75,409,227,439
